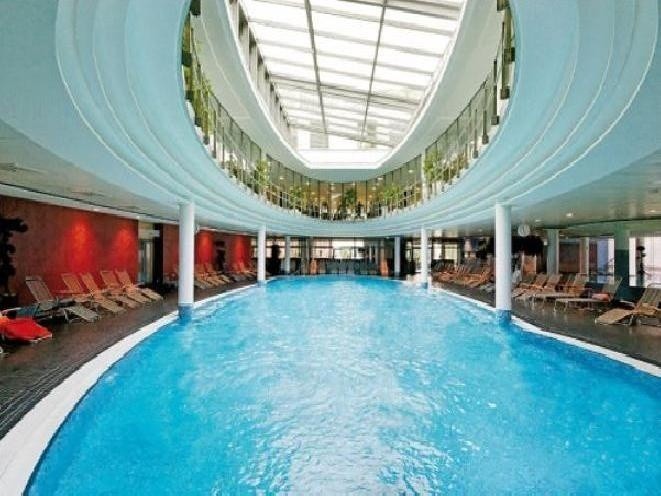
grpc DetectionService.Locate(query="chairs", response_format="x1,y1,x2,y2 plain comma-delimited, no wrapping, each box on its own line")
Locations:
309,258,390,276
2,257,274,352
428,260,661,330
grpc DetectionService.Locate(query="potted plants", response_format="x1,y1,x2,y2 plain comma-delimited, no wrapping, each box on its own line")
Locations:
1,212,29,319
